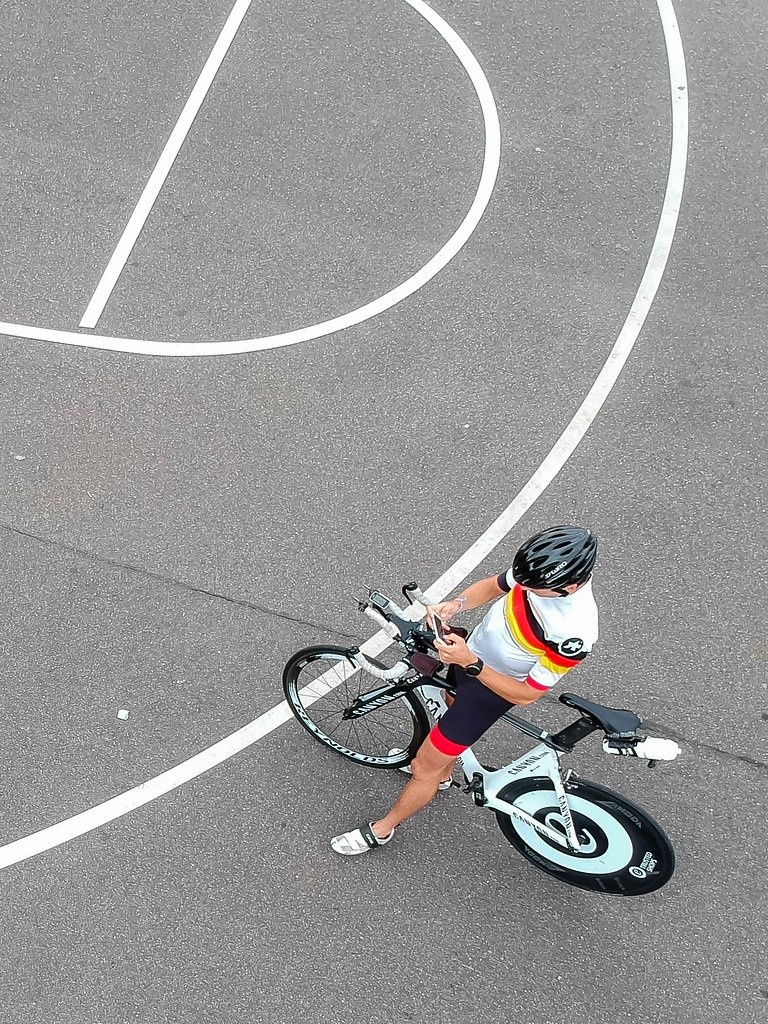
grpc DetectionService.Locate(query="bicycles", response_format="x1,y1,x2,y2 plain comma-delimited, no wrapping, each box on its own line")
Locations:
274,578,686,899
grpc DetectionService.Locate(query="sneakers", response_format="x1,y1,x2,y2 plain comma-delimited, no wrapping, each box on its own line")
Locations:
389,748,453,790
330,821,394,857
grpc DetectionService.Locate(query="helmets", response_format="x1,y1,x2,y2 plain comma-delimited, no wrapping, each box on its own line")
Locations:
511,523,599,589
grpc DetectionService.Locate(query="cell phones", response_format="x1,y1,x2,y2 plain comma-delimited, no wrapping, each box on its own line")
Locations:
431,610,449,646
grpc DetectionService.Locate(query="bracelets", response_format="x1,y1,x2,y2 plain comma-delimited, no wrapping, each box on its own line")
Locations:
454,596,466,616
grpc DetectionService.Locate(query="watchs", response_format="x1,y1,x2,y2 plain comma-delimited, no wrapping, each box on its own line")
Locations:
465,656,484,677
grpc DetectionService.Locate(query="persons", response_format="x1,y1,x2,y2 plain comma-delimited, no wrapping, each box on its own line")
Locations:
328,523,600,854
